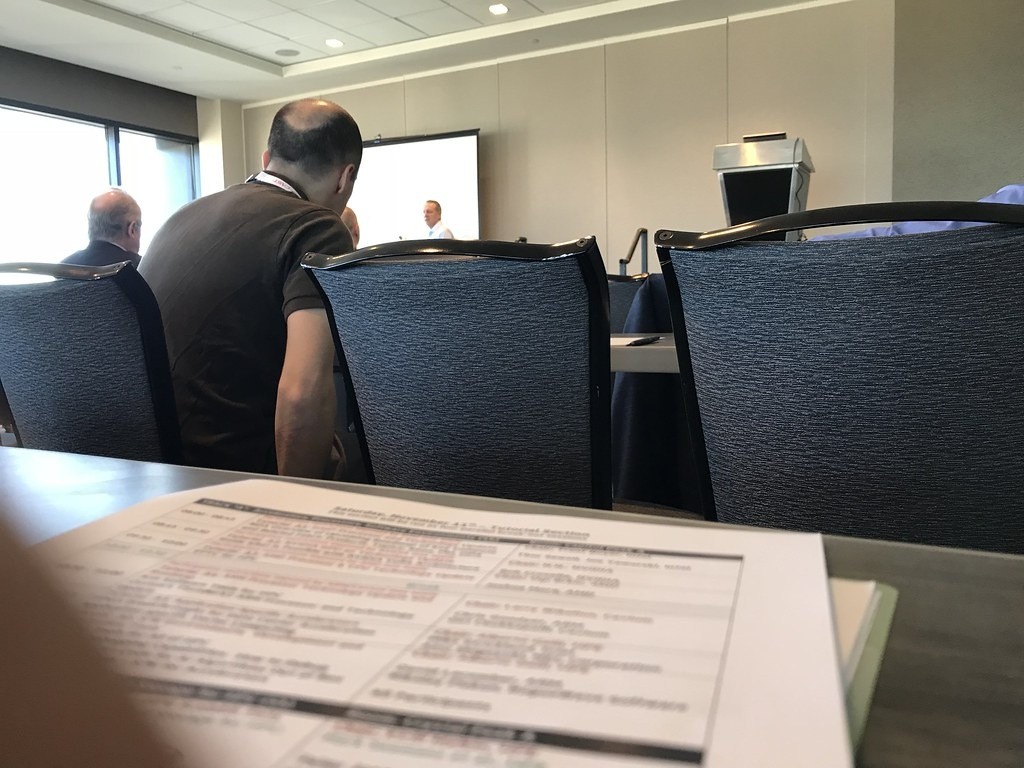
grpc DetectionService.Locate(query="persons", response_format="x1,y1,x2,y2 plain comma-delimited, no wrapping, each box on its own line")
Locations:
423,200,454,240
56,192,143,267
340,206,360,248
137,99,363,479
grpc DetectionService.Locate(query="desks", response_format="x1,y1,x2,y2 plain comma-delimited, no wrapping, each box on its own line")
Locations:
0,444,1024,768
610,333,680,373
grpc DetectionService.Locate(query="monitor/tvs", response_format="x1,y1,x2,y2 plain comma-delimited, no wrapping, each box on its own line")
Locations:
743,131,786,142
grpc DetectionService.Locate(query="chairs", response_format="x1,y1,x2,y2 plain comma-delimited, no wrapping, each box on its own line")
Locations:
299,234,612,511
608,272,650,333
0,259,185,463
654,200,1024,556
